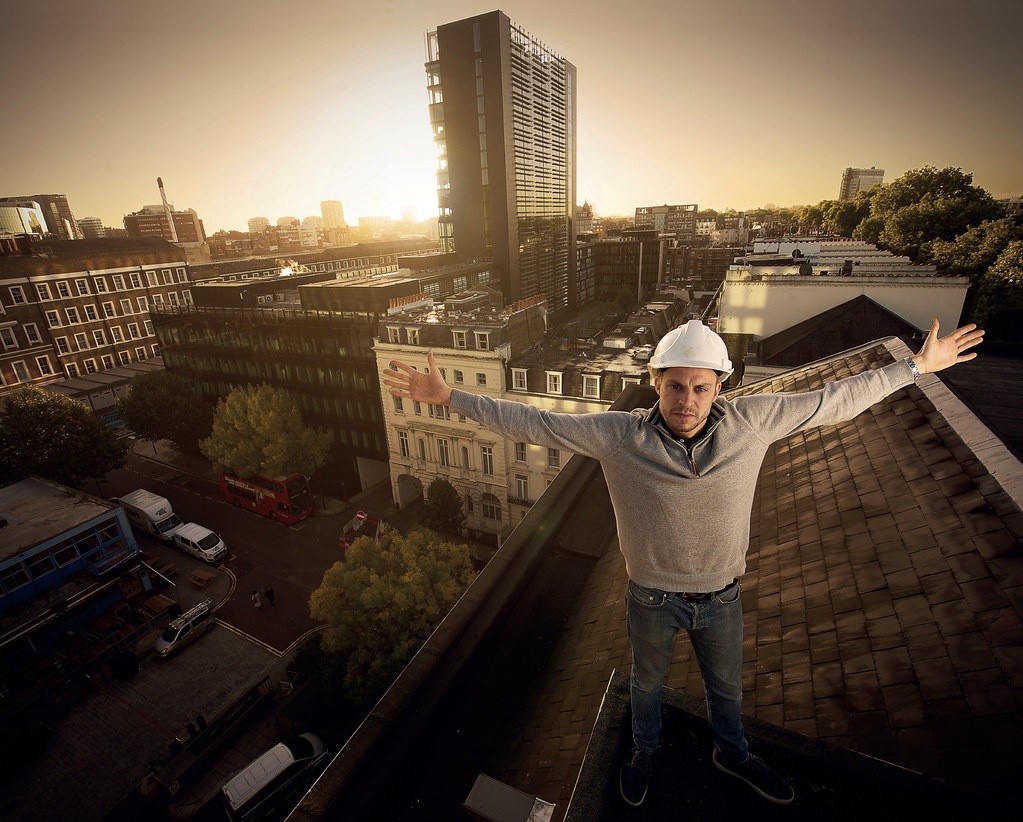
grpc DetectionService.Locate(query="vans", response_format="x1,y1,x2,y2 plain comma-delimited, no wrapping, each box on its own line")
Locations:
216,731,329,822
151,600,215,662
173,523,227,564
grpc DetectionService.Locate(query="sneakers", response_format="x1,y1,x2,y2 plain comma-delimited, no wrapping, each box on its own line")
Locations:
713,747,795,803
620,742,657,806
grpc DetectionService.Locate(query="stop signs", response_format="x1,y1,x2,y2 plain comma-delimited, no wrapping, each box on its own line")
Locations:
356,511,365,522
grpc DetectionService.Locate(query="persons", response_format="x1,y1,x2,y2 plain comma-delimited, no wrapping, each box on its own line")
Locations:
265,586,276,607
381,319,985,805
251,591,263,611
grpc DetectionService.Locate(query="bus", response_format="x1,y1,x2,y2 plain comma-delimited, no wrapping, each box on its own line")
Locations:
217,467,316,524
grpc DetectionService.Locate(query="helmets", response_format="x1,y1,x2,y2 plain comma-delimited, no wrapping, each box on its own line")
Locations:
647,320,734,383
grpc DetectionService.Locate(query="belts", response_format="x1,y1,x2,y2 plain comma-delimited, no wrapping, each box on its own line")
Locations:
679,579,739,601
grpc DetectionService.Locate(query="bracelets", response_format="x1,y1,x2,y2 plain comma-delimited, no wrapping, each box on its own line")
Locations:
904,356,921,381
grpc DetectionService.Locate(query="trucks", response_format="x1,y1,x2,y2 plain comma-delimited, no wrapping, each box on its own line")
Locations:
116,489,184,542
